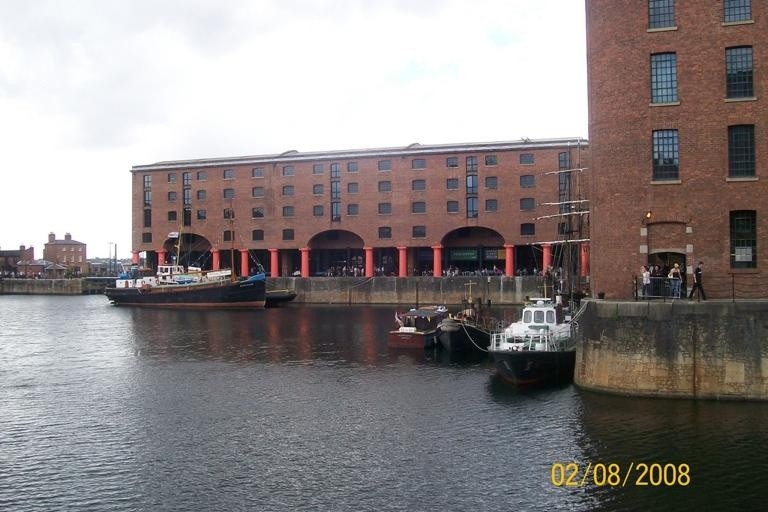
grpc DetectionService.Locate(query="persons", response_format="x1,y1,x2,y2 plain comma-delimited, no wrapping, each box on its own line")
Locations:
327,264,538,278
640,261,686,299
0,269,81,280
688,261,707,302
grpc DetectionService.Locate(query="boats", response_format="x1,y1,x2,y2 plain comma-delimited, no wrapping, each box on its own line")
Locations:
104,195,297,307
